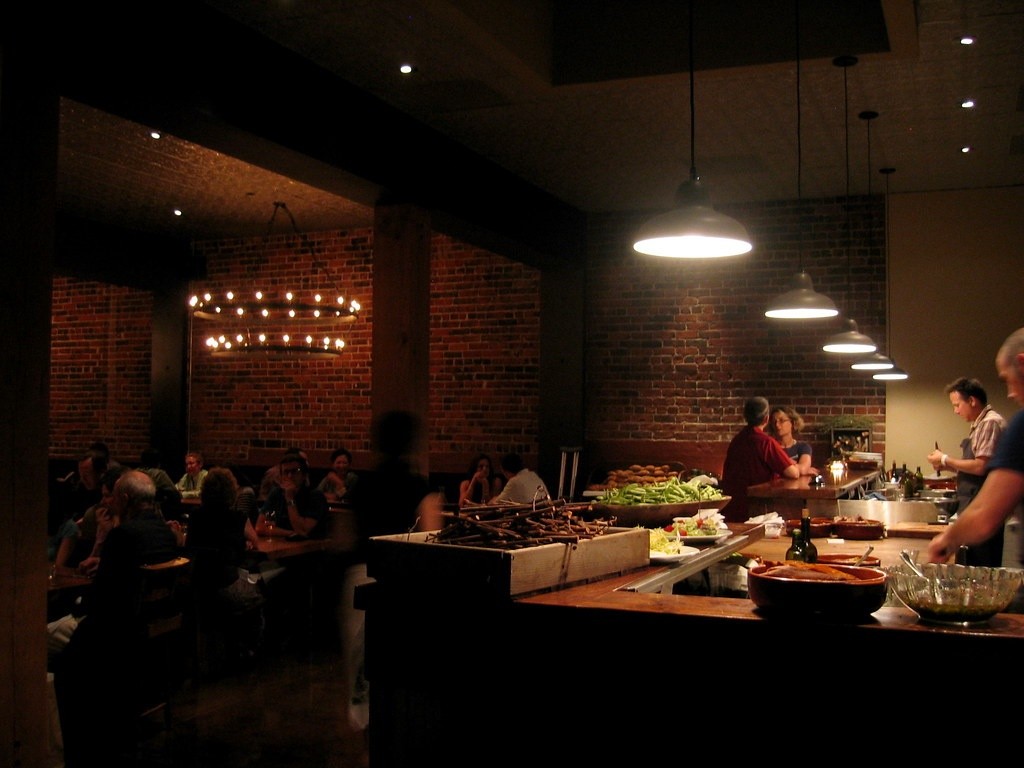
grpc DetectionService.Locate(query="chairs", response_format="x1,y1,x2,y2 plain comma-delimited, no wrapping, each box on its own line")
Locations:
136,558,206,718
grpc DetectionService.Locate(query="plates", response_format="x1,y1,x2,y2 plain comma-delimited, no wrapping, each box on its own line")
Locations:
650,546,700,562
670,530,733,542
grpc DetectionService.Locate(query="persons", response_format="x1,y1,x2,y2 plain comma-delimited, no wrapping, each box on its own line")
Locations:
336,411,444,730
928,326,1024,614
48,442,358,714
459,453,501,509
722,397,799,522
927,377,1010,567
768,405,820,476
487,453,551,505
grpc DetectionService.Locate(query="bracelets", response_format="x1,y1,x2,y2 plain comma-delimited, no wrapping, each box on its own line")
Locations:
941,455,947,466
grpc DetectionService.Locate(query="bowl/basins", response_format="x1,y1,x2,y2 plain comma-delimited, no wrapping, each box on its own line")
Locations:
832,520,883,539
816,554,881,567
884,563,1024,626
902,497,959,520
785,519,832,537
591,497,733,529
747,564,888,617
917,489,955,499
848,458,877,469
922,477,955,490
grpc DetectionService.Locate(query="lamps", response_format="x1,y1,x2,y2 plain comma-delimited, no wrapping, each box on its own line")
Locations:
765,22,909,381
634,37,754,261
189,202,361,355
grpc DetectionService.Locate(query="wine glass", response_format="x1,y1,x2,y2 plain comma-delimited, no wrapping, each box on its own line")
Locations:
265,524,273,542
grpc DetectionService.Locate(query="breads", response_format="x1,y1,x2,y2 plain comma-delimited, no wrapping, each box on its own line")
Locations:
587,464,679,490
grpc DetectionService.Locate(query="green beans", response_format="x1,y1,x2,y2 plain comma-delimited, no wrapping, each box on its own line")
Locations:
595,477,721,505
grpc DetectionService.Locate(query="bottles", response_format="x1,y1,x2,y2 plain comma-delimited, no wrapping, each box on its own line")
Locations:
264,512,271,526
892,462,922,498
785,509,817,563
180,514,189,546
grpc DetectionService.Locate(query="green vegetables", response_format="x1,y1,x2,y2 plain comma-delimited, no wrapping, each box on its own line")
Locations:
634,516,718,555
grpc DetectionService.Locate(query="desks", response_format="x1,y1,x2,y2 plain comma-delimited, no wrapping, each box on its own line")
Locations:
248,534,332,664
46,559,97,618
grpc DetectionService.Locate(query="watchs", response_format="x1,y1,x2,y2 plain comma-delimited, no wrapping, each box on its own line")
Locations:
287,500,297,505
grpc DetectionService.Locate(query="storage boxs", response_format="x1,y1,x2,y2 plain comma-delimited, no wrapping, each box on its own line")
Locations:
367,529,651,596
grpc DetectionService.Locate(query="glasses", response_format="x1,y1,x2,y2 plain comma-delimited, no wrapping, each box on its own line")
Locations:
282,465,303,475
770,418,793,424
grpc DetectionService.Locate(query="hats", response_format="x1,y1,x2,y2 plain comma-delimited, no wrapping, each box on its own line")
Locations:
745,395,768,419
